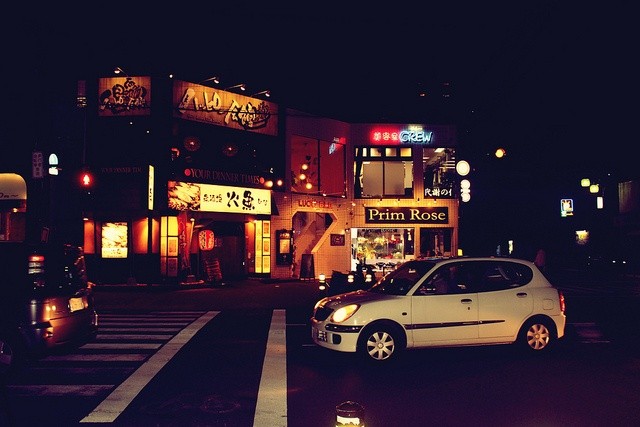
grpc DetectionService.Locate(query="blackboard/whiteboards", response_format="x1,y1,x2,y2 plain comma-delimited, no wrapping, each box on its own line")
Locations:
298,254,316,283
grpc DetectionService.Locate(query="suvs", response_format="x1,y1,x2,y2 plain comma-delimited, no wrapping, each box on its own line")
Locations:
308,254,567,369
0,241,100,377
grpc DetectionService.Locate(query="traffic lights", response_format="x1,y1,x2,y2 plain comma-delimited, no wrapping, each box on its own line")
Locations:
80,172,93,188
